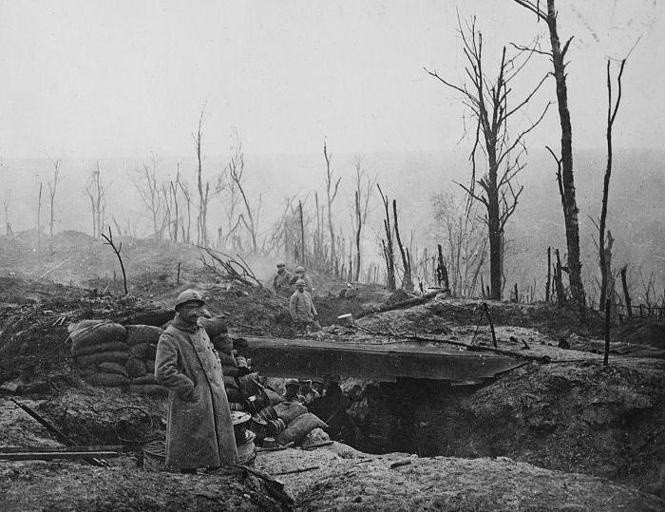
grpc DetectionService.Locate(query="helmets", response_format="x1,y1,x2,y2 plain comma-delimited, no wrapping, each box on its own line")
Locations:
175,289,205,312
295,266,304,273
285,376,324,388
276,261,285,267
295,279,305,286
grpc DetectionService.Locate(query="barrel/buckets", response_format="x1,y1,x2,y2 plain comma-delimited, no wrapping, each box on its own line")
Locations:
142,437,168,472
338,313,354,327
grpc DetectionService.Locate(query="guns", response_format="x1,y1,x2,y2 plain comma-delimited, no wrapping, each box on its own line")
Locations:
10,398,109,466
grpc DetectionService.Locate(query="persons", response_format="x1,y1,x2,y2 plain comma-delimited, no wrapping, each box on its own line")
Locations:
274,261,292,295
289,279,318,324
150,288,238,474
280,378,353,446
291,267,316,300
